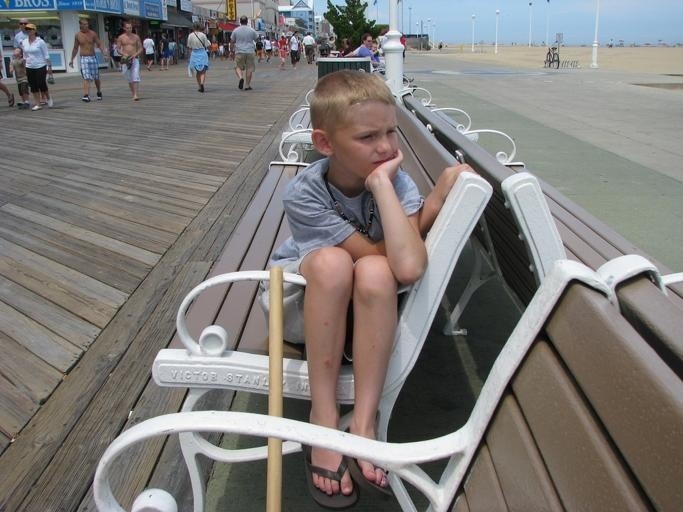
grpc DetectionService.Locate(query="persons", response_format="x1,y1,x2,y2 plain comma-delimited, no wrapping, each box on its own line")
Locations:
1,18,53,111
258,69,480,508
69,16,406,103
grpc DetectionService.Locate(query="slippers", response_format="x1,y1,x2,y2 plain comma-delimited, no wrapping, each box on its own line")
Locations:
304,444,358,509
245,86,252,90
239,78,244,89
201,84,205,93
344,426,395,505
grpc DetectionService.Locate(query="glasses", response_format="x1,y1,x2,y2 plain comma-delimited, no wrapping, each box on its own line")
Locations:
19,22,28,26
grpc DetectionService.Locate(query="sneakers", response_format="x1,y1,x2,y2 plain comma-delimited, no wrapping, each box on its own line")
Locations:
9,93,53,111
82,95,90,102
97,92,102,100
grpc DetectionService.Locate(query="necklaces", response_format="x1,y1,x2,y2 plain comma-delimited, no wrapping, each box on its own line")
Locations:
325,172,375,235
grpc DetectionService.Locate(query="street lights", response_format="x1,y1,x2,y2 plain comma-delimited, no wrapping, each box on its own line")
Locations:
528,3,533,47
471,14,476,52
547,0,552,47
494,10,500,52
408,6,440,50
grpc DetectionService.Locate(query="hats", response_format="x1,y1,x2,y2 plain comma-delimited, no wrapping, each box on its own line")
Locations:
24,24,36,30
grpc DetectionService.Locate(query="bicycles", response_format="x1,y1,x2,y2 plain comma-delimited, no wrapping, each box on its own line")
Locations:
545,46,559,68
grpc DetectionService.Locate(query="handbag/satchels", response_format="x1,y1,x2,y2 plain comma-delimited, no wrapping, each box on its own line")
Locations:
47,76,56,84
